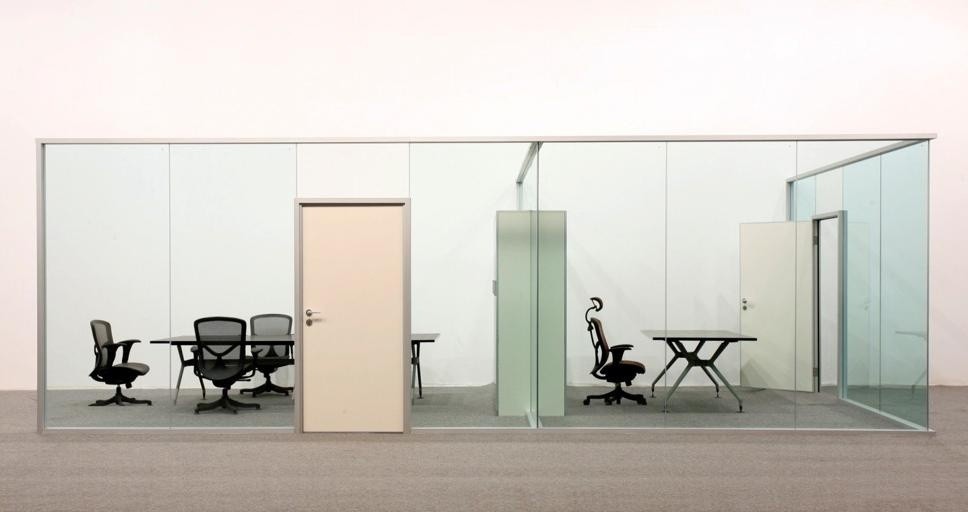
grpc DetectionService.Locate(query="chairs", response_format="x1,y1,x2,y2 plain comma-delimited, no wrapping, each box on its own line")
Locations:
583,297,647,406
88,320,152,405
240,312,294,396
191,316,261,415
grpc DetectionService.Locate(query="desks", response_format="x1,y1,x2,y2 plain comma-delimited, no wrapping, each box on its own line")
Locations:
640,329,757,412
150,332,441,404
